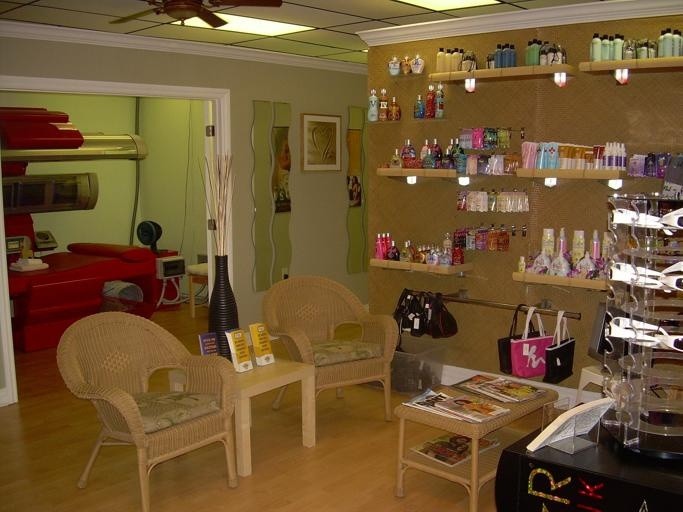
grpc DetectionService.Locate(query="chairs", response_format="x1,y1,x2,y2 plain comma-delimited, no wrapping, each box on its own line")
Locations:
13,276,103,353
57,313,236,512
262,275,399,422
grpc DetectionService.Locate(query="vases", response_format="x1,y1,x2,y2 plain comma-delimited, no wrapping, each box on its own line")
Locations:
209,255,239,362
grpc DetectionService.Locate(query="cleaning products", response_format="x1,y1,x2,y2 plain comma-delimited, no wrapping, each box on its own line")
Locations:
605,140,628,170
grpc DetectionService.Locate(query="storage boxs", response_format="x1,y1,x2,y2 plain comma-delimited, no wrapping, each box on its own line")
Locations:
358,337,445,396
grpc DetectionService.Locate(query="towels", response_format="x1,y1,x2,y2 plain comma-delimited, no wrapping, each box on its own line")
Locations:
9,259,49,272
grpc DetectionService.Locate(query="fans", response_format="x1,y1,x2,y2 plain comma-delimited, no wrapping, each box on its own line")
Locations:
110,0,282,28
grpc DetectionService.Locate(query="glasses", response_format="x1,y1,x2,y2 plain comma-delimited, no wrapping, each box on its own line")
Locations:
601,201,683,354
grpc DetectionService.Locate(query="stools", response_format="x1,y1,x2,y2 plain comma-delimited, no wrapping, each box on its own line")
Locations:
188,262,208,318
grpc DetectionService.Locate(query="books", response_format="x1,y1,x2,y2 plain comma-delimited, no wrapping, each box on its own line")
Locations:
402,374,547,468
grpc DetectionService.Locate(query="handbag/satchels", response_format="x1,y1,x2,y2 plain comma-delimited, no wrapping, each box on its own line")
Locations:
497,303,545,374
509,307,554,378
393,289,456,339
542,310,575,383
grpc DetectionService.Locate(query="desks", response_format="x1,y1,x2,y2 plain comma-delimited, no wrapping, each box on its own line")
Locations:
495,402,683,512
232,358,316,477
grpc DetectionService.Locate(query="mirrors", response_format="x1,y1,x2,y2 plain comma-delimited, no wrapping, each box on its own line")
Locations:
345,105,369,275
251,99,292,292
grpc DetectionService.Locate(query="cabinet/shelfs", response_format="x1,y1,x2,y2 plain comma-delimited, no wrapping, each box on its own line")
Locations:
368,55,682,290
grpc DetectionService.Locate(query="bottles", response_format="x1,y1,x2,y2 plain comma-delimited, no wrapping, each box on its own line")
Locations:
413,83,445,119
365,89,401,122
459,222,509,252
602,141,627,171
386,53,424,76
523,38,566,66
517,255,524,272
389,137,464,170
484,43,516,69
435,46,476,72
373,231,464,266
587,28,682,63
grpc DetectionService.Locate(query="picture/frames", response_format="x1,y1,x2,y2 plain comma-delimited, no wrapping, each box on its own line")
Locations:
300,113,343,173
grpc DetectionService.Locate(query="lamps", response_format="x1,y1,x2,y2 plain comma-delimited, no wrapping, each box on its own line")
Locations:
137,221,163,257
166,5,198,25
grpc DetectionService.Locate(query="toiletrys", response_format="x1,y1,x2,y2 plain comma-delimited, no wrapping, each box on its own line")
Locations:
556,226,568,254
525,38,568,65
575,252,594,278
531,249,550,276
367,89,379,122
550,250,570,276
588,28,682,63
626,151,681,178
391,96,401,121
486,42,517,68
374,232,465,265
390,136,458,170
521,140,604,170
413,83,445,120
378,88,389,119
436,45,478,72
589,228,601,260
